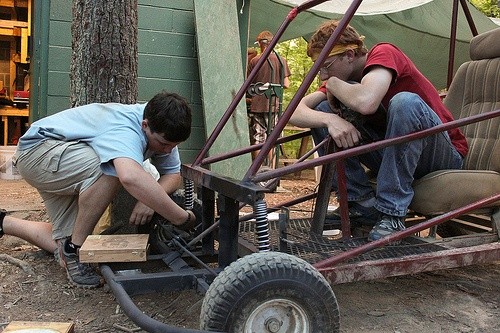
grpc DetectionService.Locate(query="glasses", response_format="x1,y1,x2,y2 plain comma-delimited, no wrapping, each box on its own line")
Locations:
319,55,340,74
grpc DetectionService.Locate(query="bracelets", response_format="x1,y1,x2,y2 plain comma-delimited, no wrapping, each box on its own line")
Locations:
171,212,191,228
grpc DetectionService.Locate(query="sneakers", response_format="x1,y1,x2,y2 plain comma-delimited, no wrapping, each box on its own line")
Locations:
53,238,104,290
367,214,406,246
323,201,379,231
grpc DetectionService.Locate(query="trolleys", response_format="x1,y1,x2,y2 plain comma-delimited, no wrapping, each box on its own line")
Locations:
248,49,290,194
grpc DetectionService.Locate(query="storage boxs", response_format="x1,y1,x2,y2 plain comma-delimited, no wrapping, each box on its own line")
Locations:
79,234,150,263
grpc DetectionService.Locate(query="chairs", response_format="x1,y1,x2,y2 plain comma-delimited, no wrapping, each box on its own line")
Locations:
367,28,500,215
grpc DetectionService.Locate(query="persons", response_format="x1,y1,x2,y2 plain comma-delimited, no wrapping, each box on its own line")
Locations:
288,21,468,246
0,91,196,290
245,31,291,169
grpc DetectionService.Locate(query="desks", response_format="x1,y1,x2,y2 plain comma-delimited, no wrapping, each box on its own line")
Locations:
0,105,29,146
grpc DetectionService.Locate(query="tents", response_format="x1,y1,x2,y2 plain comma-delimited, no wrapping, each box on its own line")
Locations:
250,0,500,92
